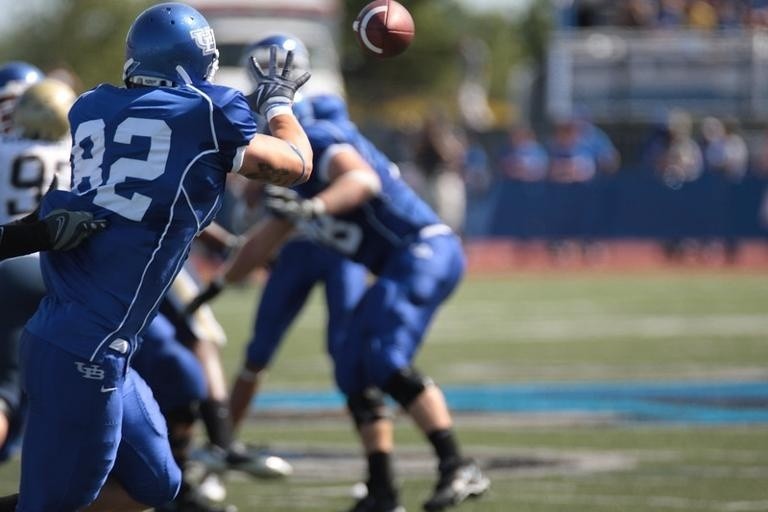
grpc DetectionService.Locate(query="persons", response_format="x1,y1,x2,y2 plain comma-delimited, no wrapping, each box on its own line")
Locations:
1,59,292,510
638,106,748,264
227,168,376,424
10,2,317,511
185,91,493,511
399,99,621,254
185,30,371,443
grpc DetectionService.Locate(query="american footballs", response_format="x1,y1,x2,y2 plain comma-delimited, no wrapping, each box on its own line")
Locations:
354,0,415,59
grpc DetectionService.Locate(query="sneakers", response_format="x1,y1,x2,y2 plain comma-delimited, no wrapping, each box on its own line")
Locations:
424,457,489,510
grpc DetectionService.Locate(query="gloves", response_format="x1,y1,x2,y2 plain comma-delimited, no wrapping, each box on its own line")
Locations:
244,44,311,115
263,184,325,235
40,209,110,252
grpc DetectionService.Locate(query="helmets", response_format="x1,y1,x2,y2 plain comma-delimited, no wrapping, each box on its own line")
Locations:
0,60,79,140
247,34,310,68
121,2,219,86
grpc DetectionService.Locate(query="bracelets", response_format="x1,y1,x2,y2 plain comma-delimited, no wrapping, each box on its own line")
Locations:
309,194,328,218
